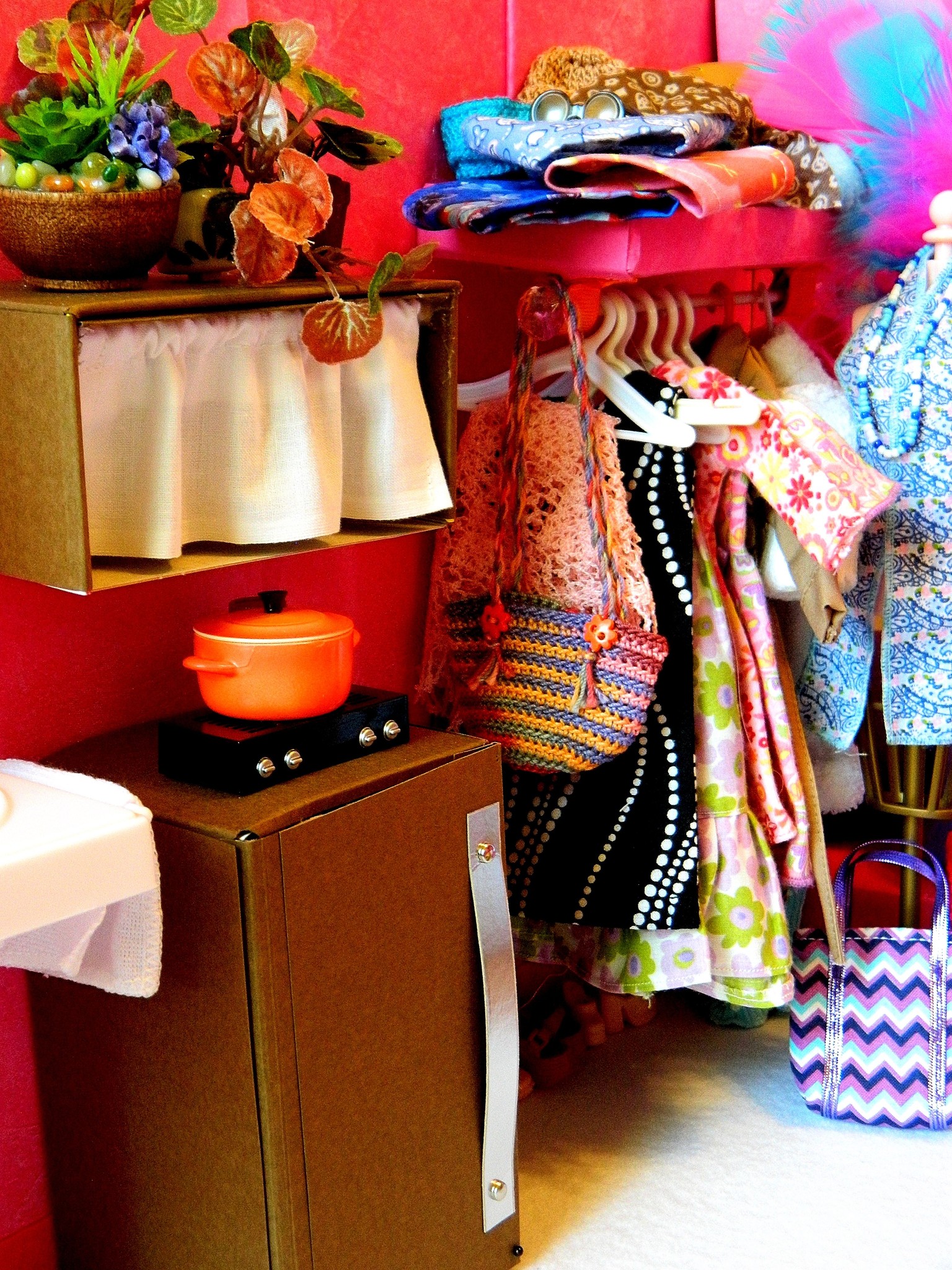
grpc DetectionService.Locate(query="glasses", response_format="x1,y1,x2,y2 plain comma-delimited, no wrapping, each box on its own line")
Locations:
528,89,624,121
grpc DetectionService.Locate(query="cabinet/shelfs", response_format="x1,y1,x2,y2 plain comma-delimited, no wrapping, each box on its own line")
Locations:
28,715,522,1270
0,277,464,594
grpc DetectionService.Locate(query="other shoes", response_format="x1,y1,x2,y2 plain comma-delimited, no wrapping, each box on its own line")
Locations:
518,981,659,1101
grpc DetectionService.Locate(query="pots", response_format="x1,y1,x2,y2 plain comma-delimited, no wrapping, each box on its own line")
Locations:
182,590,361,720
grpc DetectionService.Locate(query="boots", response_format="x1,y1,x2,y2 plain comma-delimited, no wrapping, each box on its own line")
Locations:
689,886,806,1028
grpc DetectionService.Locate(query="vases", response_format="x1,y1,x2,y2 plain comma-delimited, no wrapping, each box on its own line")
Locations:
2,187,185,295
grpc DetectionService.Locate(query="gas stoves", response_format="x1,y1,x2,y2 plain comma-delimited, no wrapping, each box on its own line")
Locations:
157,684,411,797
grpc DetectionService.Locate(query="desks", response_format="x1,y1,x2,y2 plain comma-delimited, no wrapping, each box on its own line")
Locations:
0,757,163,1270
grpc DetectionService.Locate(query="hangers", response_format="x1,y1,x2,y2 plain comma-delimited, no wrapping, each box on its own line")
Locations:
452,280,774,447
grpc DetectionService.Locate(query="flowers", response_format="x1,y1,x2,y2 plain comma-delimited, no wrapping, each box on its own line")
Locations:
1,0,441,364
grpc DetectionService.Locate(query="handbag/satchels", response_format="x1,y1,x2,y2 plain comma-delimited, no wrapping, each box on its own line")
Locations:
444,587,668,777
789,838,952,1132
